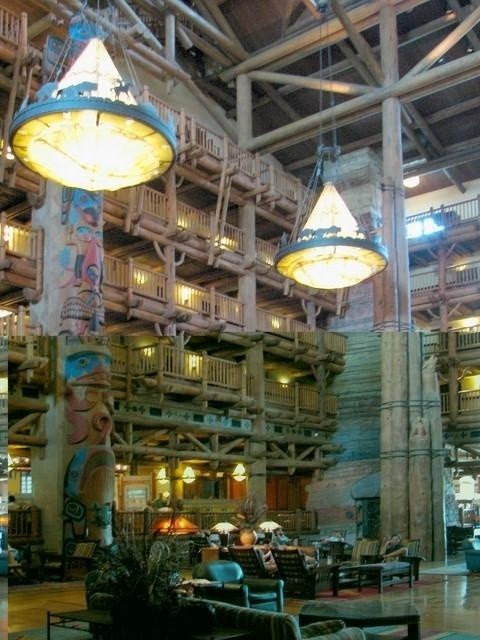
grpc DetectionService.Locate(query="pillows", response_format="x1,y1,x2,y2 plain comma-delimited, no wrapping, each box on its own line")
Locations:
300,620,346,639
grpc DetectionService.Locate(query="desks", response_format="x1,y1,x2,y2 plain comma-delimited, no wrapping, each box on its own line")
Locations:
47,607,256,640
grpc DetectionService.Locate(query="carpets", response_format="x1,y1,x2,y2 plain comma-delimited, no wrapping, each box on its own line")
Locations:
8,619,480,640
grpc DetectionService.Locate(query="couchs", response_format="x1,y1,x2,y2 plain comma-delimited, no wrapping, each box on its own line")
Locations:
89,592,367,640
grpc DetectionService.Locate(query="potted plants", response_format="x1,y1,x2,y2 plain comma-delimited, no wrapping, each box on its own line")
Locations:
235,494,268,546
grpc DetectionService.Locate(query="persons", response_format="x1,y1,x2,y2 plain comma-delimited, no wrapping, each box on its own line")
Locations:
7,494,20,510
273,527,313,563
377,535,408,565
321,531,345,544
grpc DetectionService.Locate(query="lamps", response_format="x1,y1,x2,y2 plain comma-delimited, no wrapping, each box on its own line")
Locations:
8,0,178,192
274,0,389,290
156,467,170,485
210,521,239,552
232,464,248,482
257,521,283,545
181,464,196,483
